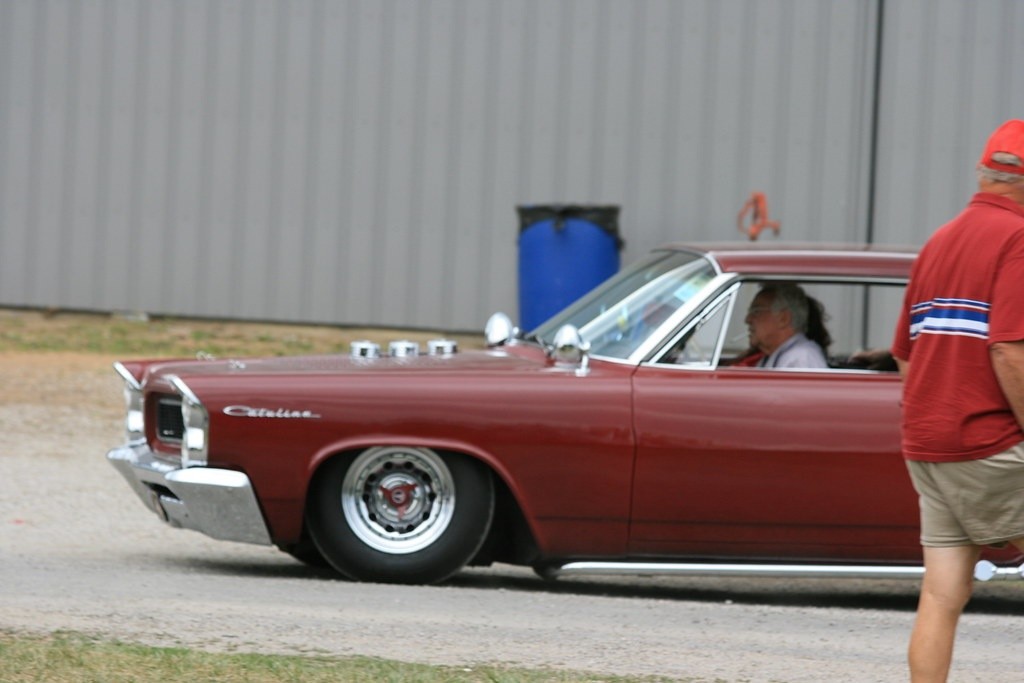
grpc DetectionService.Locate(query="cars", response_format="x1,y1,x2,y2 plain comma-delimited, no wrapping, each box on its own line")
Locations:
102,236,1024,588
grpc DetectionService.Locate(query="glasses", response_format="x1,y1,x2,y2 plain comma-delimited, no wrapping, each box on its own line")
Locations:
749,306,776,317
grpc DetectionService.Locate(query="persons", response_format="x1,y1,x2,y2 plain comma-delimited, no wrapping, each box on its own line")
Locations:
728,283,835,369
891,118,1024,683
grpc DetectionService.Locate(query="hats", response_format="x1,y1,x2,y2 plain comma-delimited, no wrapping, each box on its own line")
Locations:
980,119,1024,175
